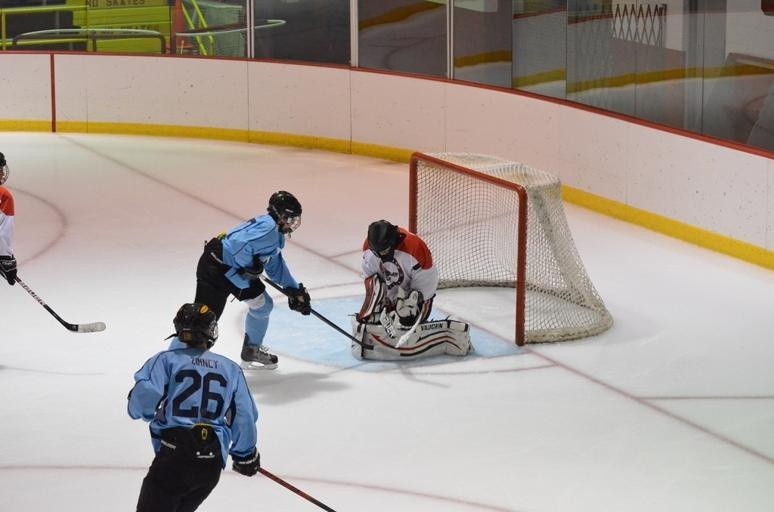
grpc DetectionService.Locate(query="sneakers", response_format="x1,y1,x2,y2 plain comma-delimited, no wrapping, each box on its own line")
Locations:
239,344,280,370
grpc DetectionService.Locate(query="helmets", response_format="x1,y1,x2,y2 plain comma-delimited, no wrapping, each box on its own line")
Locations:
268,190,302,233
367,220,401,259
173,302,219,347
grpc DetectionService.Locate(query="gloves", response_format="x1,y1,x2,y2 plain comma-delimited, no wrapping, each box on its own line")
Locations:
0,254,21,287
288,282,312,317
242,259,264,279
232,449,262,477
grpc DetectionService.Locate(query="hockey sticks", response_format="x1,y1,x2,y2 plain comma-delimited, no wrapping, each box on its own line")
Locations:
13,272,107,333
251,272,401,360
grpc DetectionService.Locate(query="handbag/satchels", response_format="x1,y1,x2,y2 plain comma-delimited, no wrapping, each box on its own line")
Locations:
175,424,223,471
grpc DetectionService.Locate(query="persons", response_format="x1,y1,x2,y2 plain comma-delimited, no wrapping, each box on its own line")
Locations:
353,220,472,361
0,151,17,285
167,190,311,370
127,303,261,512
